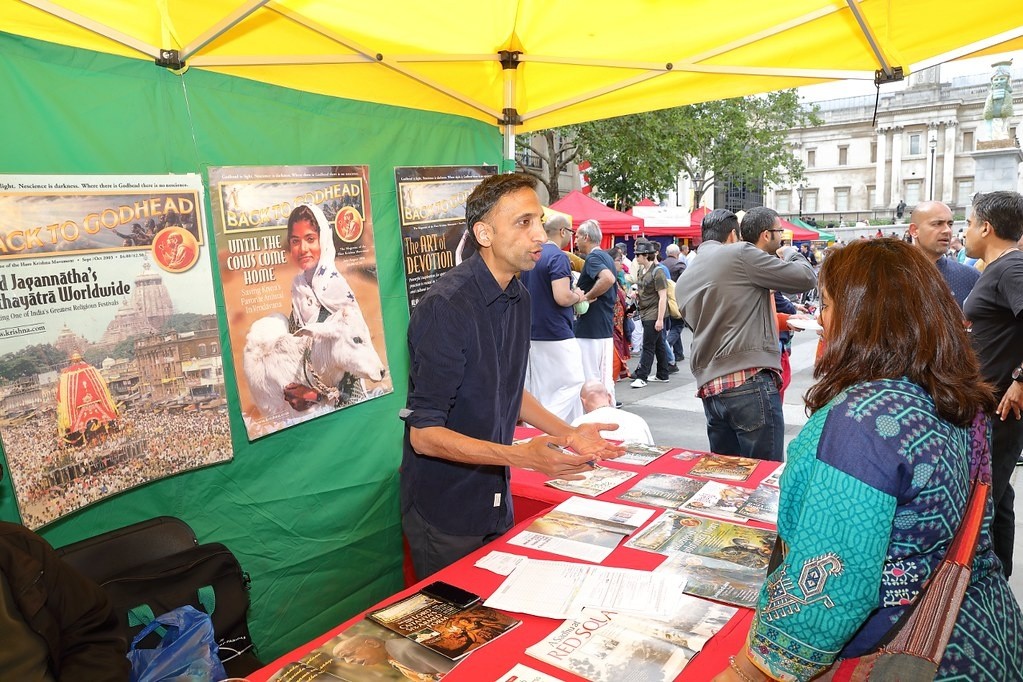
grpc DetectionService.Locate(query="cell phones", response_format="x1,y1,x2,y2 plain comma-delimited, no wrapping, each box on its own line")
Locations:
419,581,480,610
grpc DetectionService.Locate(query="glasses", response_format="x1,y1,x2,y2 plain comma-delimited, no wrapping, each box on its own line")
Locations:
636,253,643,257
766,228,784,234
560,228,576,235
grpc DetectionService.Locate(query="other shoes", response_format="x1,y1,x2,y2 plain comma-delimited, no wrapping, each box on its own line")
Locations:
616,401,623,408
1015,456,1023,466
667,362,680,373
630,350,641,357
675,354,684,360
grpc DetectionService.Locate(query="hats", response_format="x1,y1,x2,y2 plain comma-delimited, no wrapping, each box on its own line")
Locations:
634,243,657,254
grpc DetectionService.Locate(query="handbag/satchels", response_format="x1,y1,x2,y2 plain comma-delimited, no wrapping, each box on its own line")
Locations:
807,397,992,682
125,584,227,682
97,541,268,679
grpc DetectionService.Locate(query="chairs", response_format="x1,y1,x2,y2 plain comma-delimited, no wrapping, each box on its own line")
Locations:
54,517,200,585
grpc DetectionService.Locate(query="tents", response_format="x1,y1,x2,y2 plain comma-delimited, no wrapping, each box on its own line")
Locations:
540,188,836,254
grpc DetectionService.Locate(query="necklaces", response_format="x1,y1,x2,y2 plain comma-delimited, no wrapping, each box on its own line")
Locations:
590,405,615,413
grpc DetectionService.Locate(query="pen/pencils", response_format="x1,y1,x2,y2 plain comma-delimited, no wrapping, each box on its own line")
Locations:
548,441,602,469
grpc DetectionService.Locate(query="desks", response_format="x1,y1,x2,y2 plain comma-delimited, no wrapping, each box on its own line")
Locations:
231,426,784,682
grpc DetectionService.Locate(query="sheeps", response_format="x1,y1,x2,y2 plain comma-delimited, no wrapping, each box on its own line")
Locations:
242,312,387,424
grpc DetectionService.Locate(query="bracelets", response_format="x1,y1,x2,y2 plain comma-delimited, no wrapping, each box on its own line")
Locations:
575,291,581,304
728,655,753,682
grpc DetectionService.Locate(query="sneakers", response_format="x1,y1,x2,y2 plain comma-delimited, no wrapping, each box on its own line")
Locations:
630,379,647,388
647,375,669,382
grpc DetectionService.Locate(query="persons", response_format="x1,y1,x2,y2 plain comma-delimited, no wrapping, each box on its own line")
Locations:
896,200,906,219
706,236,1023,682
400,171,627,580
281,200,365,413
959,189,1023,584
333,634,451,682
519,206,1023,462
0,519,131,682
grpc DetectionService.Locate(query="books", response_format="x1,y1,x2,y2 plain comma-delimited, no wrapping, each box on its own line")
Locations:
262,443,787,682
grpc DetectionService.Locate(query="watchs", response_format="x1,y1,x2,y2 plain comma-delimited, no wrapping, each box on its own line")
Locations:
1008,363,1023,382
